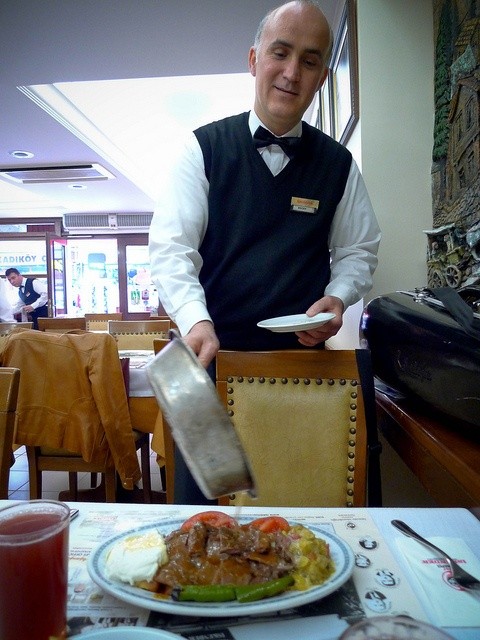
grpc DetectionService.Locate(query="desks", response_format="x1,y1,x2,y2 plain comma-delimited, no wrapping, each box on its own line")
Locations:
373,375,480,509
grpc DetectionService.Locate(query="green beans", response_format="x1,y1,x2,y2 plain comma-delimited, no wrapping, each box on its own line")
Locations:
171,573,294,602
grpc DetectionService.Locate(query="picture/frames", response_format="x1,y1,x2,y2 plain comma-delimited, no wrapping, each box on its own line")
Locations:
319,70,335,139
326,2,359,145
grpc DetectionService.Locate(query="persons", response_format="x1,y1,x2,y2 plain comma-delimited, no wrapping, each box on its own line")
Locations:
5,268,48,331
148,0,381,508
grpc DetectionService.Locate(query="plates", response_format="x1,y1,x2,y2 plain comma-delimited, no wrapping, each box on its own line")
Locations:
67,625,189,640
86,515,356,619
257,312,337,333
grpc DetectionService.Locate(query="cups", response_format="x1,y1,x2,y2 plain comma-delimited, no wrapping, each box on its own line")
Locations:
0,499,71,640
338,615,455,640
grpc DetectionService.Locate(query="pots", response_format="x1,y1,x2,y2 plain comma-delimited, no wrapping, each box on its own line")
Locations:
144,328,260,501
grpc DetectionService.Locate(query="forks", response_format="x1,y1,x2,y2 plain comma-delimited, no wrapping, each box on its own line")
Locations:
391,519,480,593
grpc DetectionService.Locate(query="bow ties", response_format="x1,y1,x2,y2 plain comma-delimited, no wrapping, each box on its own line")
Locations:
250,126,303,159
19,285,24,290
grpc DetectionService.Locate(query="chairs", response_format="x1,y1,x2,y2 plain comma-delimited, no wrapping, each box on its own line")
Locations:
85,313,123,331
18,333,150,502
109,319,170,349
0,366,20,499
37,317,85,331
152,339,175,503
214,348,370,507
1,322,33,335
